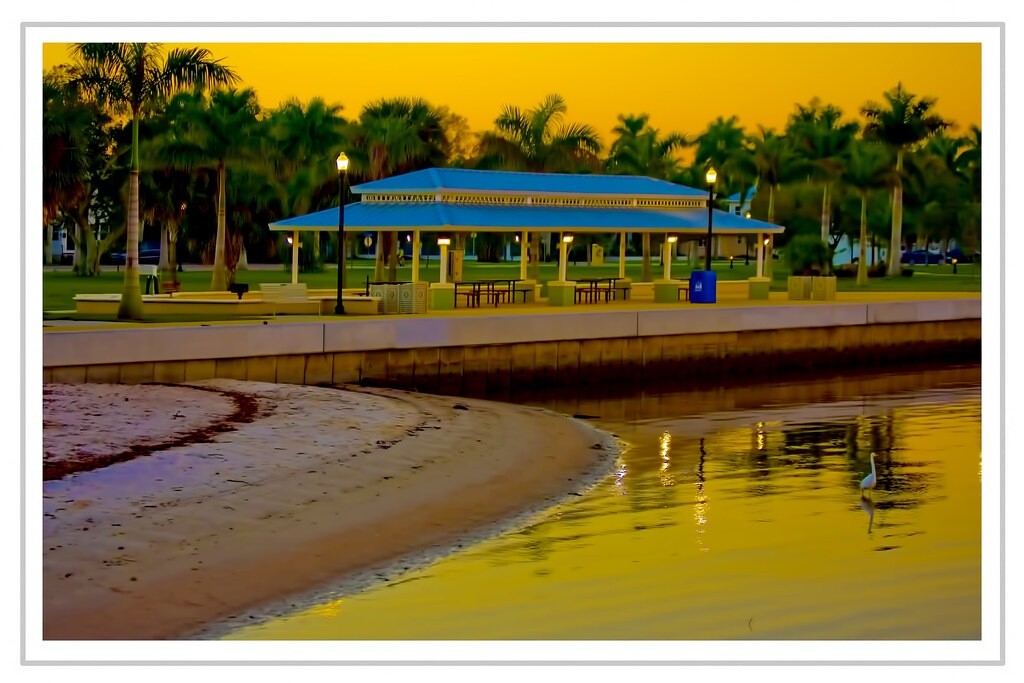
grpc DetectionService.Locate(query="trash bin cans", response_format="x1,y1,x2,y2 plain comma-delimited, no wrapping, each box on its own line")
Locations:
690,270,716,303
369,282,428,314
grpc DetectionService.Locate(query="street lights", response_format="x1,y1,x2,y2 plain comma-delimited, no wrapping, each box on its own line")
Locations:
333,150,350,316
705,165,718,272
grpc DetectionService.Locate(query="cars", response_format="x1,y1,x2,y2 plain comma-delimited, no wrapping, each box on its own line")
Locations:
900,249,952,264
947,248,981,263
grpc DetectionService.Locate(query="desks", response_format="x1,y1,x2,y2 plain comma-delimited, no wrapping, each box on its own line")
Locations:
573,278,624,304
454,279,525,308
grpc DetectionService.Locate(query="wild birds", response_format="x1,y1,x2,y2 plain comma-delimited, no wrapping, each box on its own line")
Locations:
860,494,875,535
859,452,878,494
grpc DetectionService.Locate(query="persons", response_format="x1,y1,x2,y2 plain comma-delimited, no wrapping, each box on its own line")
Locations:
397,246,404,267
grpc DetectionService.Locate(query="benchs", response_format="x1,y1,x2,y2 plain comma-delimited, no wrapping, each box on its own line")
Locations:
259,283,321,316
573,287,616,304
452,292,502,307
578,288,632,301
473,289,533,303
